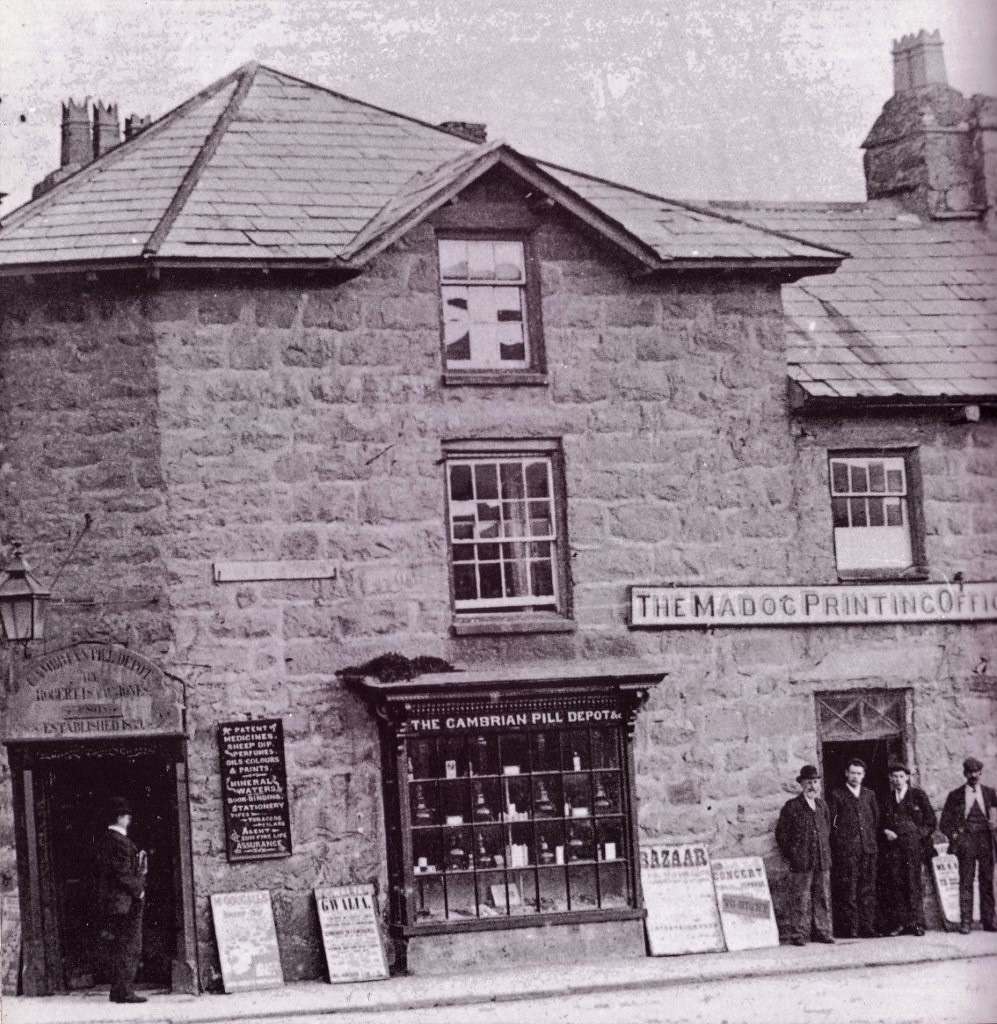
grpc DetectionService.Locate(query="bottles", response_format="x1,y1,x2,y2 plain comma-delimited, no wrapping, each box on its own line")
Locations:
533,729,614,866
414,737,494,870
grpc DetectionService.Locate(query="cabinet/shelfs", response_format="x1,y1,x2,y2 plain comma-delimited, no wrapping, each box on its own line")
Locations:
406,723,635,921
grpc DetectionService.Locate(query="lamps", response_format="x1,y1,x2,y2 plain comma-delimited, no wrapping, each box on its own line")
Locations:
0,539,52,660
947,404,981,427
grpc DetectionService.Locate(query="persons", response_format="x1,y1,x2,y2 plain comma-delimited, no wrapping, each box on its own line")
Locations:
827,758,883,940
775,764,836,948
882,762,937,940
94,795,149,1005
937,757,997,937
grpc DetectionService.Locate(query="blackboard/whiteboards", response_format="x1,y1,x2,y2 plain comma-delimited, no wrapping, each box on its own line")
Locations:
639,842,727,956
931,843,983,933
313,883,390,984
710,856,780,952
216,718,293,864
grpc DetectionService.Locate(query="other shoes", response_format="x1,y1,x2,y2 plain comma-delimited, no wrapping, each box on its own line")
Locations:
889,925,908,936
850,932,858,938
811,935,834,943
864,932,883,937
983,924,997,932
915,925,924,936
959,923,971,934
793,936,806,946
109,994,147,1003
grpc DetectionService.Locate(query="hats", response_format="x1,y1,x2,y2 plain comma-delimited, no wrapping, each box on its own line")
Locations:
963,758,983,771
796,765,822,783
102,797,133,814
